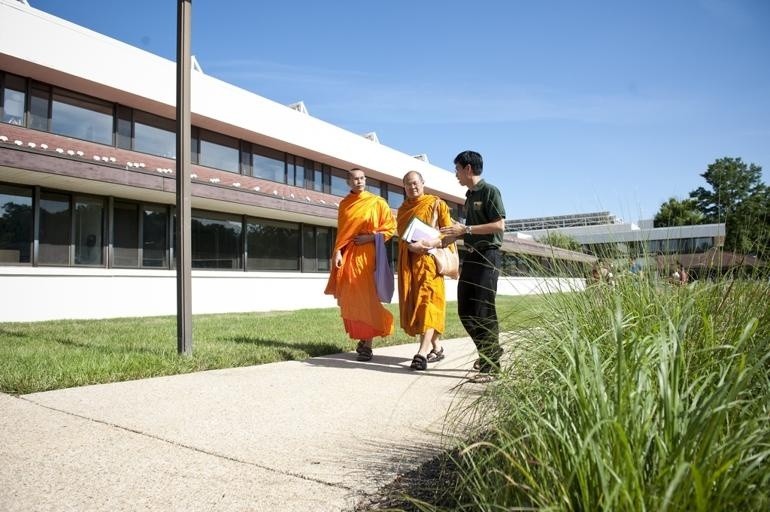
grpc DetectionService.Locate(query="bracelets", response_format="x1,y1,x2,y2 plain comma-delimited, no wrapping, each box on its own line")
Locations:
465,225,472,233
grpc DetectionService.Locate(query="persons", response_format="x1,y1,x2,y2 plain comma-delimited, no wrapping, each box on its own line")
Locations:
324,168,396,361
592,259,608,281
440,150,505,382
396,171,455,370
623,255,643,279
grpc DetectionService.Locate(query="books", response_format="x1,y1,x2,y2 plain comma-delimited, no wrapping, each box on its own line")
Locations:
402,218,440,255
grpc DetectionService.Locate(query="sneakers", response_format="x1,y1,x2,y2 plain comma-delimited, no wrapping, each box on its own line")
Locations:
474,348,503,372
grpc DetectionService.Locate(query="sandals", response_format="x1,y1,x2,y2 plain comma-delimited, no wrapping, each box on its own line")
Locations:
408,346,445,371
356,340,373,361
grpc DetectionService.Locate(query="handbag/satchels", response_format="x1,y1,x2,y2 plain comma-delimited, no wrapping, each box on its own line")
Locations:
428,248,459,279
